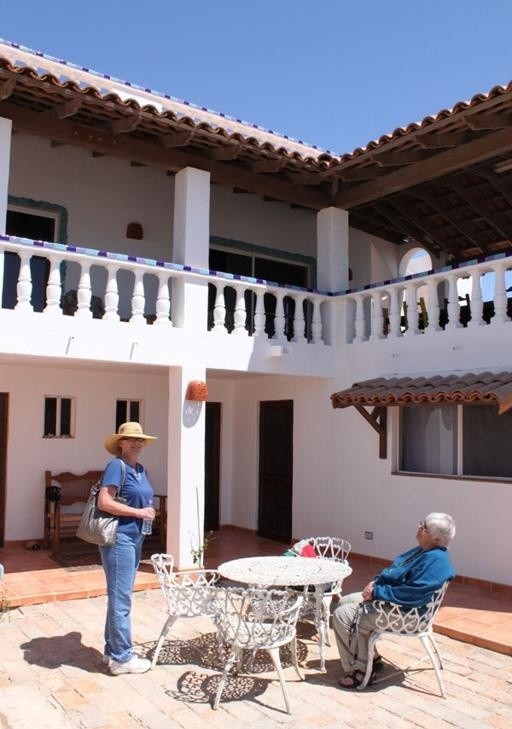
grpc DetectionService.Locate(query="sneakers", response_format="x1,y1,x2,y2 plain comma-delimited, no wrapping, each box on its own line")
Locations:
102,654,152,675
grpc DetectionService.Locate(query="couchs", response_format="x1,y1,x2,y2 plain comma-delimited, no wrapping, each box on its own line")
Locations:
45,470,167,556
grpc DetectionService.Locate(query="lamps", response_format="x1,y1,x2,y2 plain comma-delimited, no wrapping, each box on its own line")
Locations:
185,379,209,401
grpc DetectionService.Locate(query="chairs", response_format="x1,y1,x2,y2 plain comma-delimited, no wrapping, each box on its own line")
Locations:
150,553,231,669
205,587,306,715
356,580,450,699
259,537,354,671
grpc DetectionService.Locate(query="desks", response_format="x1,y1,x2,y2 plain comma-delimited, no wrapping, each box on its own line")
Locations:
217,556,352,676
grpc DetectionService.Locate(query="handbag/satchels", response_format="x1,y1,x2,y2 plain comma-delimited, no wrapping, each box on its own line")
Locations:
75,485,128,547
282,540,333,591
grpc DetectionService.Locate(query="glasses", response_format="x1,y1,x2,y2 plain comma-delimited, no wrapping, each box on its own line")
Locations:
418,520,429,534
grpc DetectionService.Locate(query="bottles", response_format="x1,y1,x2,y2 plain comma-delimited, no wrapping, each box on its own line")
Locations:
141,499,153,535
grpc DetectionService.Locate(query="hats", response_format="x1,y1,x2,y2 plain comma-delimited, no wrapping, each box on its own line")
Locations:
104,421,158,456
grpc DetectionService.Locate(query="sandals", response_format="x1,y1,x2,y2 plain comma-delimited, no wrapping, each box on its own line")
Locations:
338,655,382,688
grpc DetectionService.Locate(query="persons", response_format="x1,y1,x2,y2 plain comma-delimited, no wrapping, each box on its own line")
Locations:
327,509,456,690
93,420,157,676
